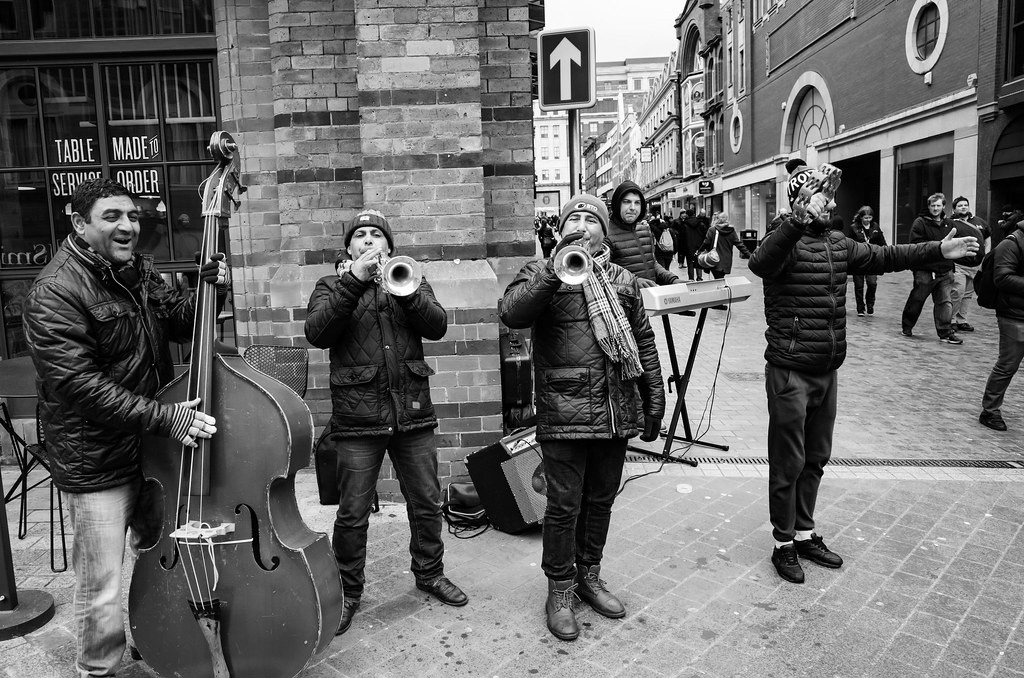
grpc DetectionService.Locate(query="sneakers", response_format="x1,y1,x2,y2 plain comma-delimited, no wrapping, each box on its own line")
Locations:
952,322,974,332
901,328,912,336
939,334,963,344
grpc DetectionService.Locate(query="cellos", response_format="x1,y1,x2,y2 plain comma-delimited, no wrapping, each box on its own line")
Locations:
126,131,347,678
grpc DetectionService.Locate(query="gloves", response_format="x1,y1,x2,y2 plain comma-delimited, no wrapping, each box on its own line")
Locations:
193,252,230,314
640,416,661,442
556,233,584,255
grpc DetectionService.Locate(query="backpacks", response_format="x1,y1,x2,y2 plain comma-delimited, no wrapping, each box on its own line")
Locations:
657,228,673,252
315,416,380,514
973,234,1024,310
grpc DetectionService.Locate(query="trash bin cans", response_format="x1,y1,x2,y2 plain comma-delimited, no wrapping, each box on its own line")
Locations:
740,229,758,259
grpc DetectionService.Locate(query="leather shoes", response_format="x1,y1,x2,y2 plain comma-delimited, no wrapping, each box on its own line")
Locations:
336,602,361,634
771,543,805,584
979,409,1007,431
416,576,468,606
794,533,843,569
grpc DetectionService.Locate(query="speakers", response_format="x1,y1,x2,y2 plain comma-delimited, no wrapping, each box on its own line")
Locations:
463,425,549,535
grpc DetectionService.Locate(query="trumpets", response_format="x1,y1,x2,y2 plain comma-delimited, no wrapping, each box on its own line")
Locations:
373,250,422,297
554,238,594,285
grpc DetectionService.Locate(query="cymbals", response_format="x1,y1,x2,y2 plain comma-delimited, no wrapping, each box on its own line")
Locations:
792,162,842,225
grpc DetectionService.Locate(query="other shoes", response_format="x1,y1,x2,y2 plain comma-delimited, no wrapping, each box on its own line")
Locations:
857,309,865,316
867,304,874,315
130,645,144,660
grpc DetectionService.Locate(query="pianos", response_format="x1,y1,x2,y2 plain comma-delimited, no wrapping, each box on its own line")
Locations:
626,275,752,469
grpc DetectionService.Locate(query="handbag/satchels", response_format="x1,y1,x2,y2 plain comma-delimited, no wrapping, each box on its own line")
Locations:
441,481,486,527
698,230,720,268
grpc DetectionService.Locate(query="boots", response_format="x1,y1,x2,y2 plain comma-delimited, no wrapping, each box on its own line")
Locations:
576,564,626,618
545,576,579,639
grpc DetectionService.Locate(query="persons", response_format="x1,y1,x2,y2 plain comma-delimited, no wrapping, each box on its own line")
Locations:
848,205,888,316
901,193,963,344
978,220,1024,431
949,196,992,333
303,208,469,636
501,193,666,641
20,180,231,678
747,158,980,584
998,209,1024,241
647,205,751,311
603,179,693,435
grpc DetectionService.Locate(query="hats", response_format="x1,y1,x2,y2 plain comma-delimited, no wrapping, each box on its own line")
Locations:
715,212,729,223
558,194,609,237
344,209,393,257
785,158,819,212
